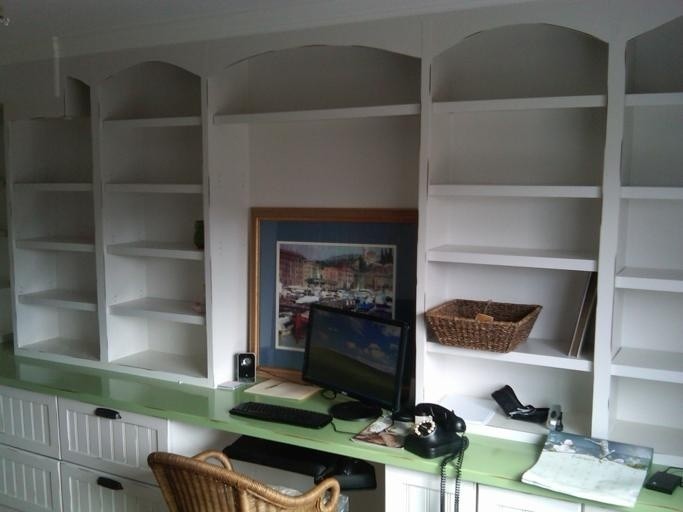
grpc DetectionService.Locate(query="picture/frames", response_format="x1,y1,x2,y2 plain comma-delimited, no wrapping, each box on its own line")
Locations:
247,206,418,384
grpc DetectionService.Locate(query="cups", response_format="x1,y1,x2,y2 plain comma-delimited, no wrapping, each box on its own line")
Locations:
194,220,205,250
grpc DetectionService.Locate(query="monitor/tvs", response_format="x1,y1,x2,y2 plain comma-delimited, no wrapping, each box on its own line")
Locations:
301,302,409,421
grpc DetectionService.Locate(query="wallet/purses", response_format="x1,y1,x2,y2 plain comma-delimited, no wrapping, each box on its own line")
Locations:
491,384,549,423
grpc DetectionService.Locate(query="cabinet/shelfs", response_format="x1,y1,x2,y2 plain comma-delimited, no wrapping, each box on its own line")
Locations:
203,37,425,129
1,388,219,512
413,2,682,468
385,467,625,511
3,43,253,389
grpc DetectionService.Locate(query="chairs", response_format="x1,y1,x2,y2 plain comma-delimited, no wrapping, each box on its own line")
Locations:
146,449,342,512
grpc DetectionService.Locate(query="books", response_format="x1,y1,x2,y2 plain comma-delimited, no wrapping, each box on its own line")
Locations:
567,272,597,359
517,427,656,510
242,378,321,401
350,414,415,453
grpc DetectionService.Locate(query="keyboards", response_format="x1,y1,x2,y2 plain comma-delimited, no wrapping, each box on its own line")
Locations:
229,400,333,429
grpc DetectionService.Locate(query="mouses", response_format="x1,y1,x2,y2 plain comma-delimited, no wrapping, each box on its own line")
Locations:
368,422,389,434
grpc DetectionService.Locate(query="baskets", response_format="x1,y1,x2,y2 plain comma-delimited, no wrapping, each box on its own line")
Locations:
424,298,543,353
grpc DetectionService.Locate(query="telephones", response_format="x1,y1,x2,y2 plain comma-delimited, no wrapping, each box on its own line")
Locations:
403,403,469,463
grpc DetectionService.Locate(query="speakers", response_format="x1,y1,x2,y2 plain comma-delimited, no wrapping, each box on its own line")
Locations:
236,352,257,384
